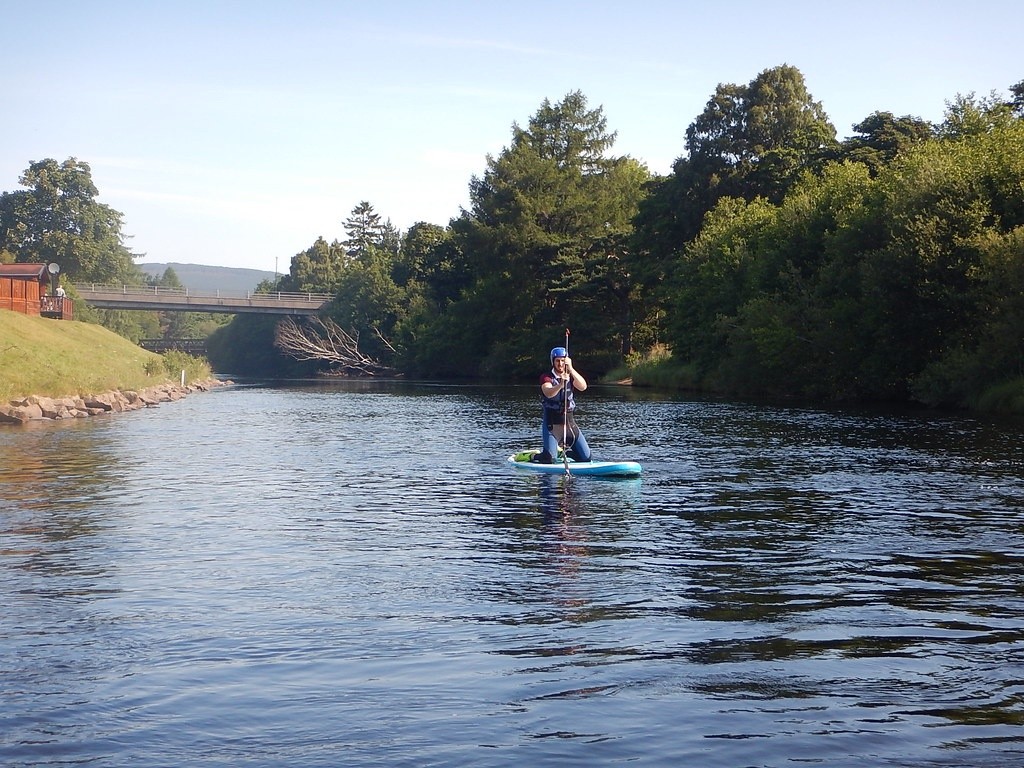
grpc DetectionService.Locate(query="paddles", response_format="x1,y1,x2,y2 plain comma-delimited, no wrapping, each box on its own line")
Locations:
565,327,571,477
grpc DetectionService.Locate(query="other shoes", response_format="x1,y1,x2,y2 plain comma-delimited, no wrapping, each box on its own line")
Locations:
515,453,535,462
557,446,563,457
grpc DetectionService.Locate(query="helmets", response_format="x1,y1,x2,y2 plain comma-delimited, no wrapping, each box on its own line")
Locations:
550,346,569,367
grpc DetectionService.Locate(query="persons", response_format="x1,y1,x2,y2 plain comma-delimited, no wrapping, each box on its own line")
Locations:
41,285,66,311
514,347,591,465
